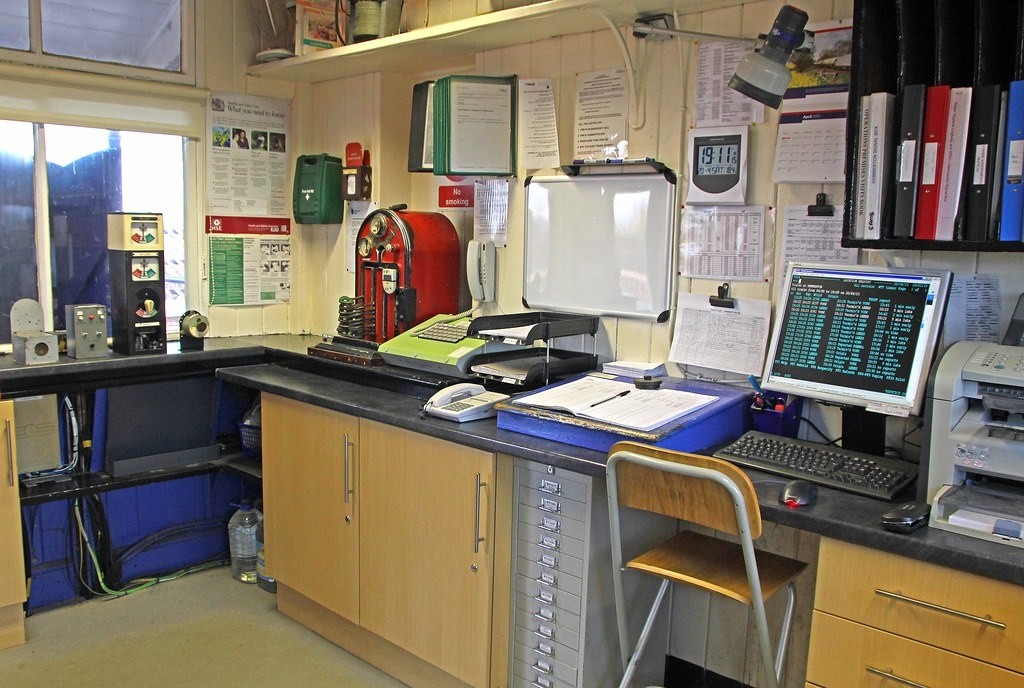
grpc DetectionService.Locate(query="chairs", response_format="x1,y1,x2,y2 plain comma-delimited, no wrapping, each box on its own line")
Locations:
606,441,809,688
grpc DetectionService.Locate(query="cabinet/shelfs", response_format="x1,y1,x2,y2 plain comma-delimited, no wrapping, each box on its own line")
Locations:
466,311,599,387
1,347,1024,688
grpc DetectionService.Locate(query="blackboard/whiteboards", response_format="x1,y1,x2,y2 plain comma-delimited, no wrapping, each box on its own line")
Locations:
521,172,673,323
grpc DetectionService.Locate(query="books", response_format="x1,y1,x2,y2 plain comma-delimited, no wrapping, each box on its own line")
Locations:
512,375,720,432
935,87,972,241
848,92,895,240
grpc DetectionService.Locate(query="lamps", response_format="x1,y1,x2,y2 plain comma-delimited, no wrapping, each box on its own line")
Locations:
631,5,809,110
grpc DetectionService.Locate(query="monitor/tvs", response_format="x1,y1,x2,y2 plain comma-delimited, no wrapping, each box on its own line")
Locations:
759,261,955,459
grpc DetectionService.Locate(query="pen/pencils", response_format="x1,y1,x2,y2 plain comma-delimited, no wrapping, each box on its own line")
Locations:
590,389,630,408
748,374,762,393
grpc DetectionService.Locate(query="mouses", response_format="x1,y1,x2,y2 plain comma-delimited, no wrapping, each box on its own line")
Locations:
779,480,818,506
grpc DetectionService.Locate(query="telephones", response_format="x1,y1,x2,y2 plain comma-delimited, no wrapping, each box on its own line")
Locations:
423,383,510,423
466,239,495,302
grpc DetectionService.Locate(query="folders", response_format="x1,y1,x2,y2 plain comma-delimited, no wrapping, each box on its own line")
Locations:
895,80,1024,240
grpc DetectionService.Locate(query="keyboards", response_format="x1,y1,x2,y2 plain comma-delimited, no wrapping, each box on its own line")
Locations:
713,430,919,503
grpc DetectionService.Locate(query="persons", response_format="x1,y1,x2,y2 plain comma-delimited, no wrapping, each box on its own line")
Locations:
232,129,265,149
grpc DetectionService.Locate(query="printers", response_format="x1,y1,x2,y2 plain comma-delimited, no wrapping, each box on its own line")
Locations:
921,292,1024,550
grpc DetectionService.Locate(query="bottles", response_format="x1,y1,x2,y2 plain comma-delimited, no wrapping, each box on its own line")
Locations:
227,500,263,584
254,516,279,594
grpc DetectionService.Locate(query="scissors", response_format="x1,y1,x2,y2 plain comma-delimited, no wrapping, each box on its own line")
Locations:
752,391,785,410
